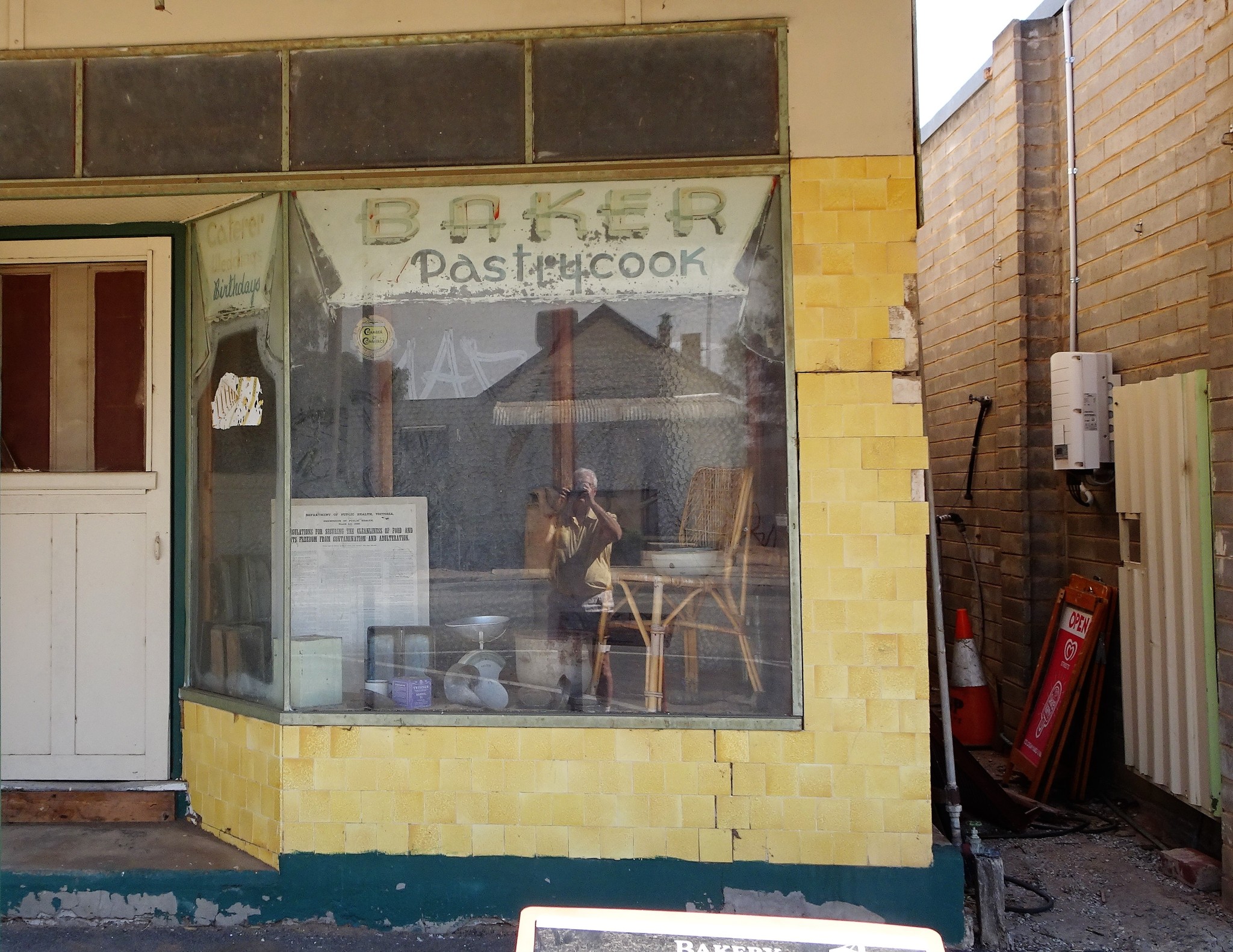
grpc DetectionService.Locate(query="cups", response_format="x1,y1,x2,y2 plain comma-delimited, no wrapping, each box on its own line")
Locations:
365,679,392,697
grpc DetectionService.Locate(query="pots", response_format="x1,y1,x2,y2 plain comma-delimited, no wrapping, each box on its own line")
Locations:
515,619,593,698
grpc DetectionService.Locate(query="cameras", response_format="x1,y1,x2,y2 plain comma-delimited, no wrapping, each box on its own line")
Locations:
564,490,587,502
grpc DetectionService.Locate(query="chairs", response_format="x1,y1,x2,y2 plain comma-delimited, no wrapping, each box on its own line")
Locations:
584,466,768,714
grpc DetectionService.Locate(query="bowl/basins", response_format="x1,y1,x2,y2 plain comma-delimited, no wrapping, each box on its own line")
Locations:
655,546,719,578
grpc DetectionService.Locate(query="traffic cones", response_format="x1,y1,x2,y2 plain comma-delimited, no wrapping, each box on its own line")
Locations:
946,607,998,766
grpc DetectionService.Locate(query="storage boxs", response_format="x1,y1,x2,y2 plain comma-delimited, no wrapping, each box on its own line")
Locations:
273,635,342,706
391,675,432,708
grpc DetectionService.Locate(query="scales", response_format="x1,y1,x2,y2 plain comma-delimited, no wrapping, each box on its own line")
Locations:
439,614,514,681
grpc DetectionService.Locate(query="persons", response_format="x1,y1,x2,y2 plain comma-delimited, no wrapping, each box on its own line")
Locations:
542,468,623,714
